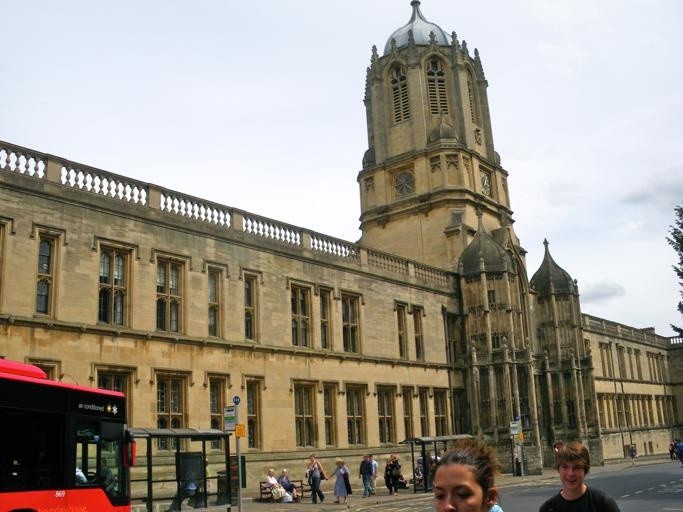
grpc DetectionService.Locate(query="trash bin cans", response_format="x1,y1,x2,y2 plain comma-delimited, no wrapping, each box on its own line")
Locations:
217,470,227,505
516,459,528,475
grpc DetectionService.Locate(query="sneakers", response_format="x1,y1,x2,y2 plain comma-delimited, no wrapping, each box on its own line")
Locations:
293,497,297,500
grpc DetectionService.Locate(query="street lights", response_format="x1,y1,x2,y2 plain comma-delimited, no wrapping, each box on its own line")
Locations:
655,351,675,444
607,341,637,459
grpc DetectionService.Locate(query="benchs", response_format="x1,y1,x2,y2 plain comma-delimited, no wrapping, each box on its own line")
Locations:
260,480,311,501
131,486,230,507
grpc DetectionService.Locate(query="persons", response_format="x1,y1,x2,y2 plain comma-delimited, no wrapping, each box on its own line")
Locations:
267,453,443,504
538,440,620,511
668,438,682,467
628,444,638,464
432,437,502,511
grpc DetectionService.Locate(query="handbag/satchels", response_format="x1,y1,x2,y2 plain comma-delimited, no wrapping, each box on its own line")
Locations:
271,486,282,500
320,471,324,480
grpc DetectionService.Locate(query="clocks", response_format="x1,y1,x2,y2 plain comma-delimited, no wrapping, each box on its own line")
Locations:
478,170,490,196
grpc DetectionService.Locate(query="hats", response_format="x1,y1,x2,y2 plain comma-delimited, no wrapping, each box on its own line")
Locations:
335,459,344,465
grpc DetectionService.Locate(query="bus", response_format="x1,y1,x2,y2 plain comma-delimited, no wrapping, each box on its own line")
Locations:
0,357,135,511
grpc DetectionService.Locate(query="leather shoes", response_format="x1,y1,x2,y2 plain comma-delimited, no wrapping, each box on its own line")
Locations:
334,501,339,503
